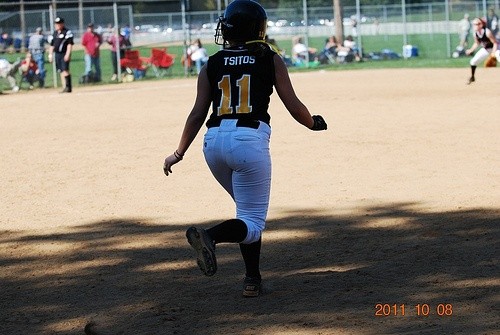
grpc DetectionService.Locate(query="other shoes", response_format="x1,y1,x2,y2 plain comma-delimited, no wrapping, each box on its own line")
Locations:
185,226,218,277
241,277,262,297
466,77,475,84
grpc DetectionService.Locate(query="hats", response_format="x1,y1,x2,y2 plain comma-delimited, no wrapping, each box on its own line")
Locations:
54,17,65,24
87,22,94,27
470,18,483,25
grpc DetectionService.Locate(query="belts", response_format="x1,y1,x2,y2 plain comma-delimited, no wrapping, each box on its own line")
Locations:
208,115,260,129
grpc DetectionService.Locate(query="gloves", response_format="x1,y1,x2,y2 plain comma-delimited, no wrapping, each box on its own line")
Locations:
311,114,328,130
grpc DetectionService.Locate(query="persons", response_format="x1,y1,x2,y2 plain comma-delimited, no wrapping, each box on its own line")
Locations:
487,8,499,37
465,18,500,85
0,26,50,93
265,34,368,67
187,38,209,74
104,25,126,80
81,22,103,82
98,24,132,49
164,0,327,297
456,13,471,46
48,17,74,93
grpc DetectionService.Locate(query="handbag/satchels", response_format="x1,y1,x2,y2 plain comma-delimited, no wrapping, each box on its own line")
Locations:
181,53,191,67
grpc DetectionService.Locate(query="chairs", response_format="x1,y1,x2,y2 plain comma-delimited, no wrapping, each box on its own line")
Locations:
121,47,176,79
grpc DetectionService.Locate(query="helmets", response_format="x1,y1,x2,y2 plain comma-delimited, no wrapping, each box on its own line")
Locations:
222,0,267,41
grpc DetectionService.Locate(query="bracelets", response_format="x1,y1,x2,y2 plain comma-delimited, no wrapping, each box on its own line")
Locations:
174,150,183,160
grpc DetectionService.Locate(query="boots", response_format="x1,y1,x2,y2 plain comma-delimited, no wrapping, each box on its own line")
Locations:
61,74,71,93
